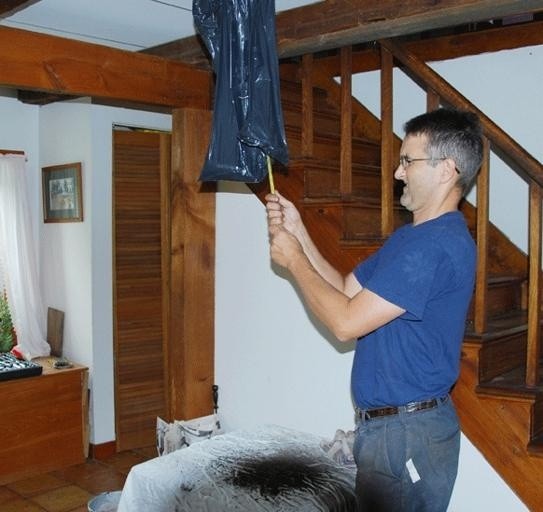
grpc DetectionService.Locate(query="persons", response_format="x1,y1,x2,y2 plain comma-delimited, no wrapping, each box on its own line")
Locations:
264,107,486,511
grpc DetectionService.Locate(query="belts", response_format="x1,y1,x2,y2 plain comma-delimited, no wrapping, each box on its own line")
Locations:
360,397,446,420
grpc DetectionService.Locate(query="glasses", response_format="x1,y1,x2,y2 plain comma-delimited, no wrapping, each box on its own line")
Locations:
399,156,461,175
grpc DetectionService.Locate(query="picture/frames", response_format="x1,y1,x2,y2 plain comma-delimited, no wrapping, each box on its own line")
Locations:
40,161,82,223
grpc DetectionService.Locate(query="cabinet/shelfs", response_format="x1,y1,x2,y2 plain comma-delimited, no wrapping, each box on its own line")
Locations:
1,355,91,487
